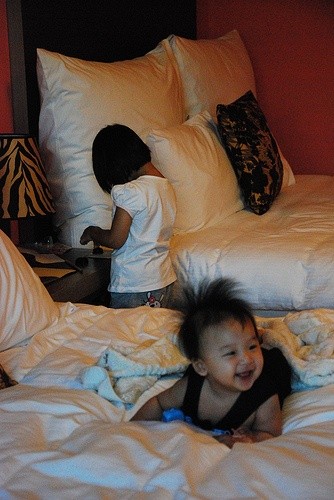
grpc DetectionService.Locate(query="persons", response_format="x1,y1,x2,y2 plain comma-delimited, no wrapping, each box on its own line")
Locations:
129,278,293,448
79,123,178,310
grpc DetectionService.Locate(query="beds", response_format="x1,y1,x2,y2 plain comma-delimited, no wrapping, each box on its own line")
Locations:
7,0,334,309
0,228,334,499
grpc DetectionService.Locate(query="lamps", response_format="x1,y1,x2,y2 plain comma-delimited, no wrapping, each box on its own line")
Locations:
0,133,56,246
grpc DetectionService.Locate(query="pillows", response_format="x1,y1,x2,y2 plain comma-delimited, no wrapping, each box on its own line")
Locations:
37,29,297,250
0,228,60,352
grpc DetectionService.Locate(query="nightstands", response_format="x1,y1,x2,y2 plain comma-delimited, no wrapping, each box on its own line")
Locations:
17,244,112,308
